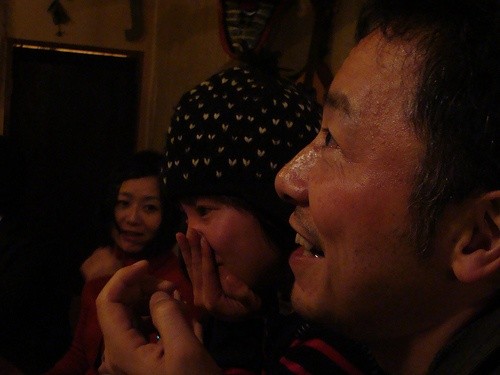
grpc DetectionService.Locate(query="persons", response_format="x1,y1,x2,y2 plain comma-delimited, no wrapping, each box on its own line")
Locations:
43,146,203,374
88,0,500,374
153,63,387,373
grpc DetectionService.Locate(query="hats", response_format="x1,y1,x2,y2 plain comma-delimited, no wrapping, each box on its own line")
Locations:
161,64,323,221
